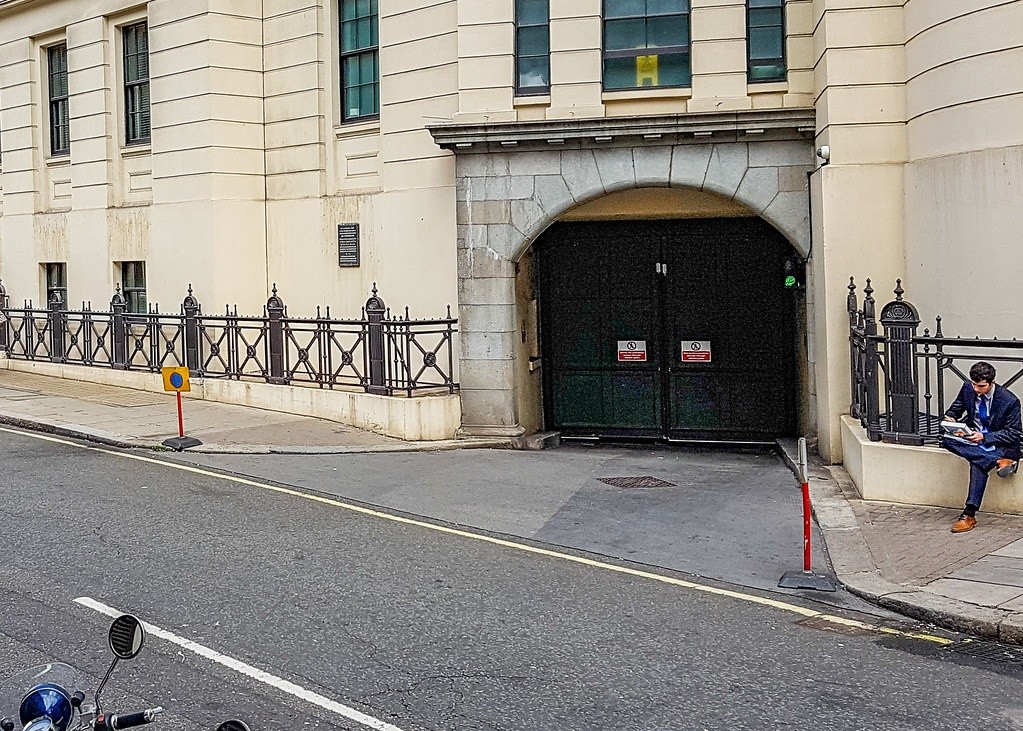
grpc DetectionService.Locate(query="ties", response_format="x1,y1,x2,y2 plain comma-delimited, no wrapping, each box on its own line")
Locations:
978,395,987,430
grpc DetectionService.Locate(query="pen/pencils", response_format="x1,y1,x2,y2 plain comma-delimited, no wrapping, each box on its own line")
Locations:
944,414,951,421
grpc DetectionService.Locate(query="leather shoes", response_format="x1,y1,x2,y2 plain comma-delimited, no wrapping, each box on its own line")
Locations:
996,459,1017,478
951,515,978,533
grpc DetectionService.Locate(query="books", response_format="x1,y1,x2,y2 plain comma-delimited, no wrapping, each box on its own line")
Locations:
941,421,973,436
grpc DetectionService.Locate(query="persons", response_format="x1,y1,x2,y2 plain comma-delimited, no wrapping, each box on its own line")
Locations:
940,361,1023,533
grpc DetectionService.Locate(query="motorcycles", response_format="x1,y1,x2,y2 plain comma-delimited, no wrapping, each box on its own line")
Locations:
0,614,251,731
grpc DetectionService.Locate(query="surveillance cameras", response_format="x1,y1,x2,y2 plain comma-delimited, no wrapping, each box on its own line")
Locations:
816,145,829,159
